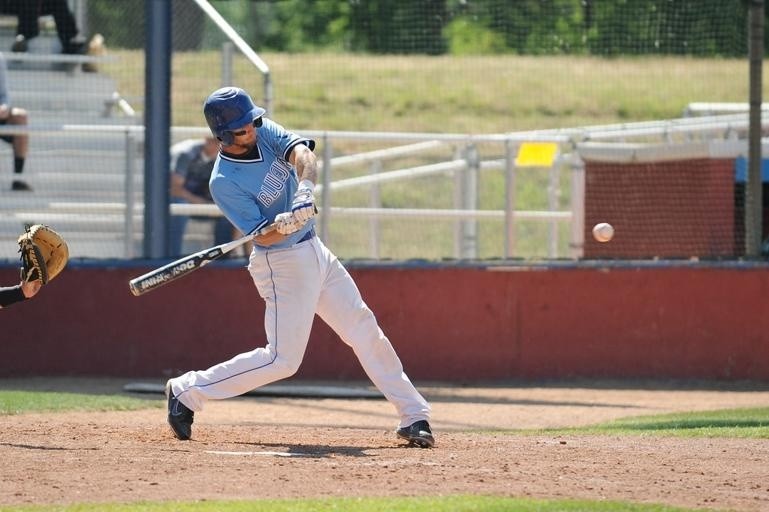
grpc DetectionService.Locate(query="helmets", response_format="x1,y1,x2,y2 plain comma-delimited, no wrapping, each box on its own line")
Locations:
204,86,265,147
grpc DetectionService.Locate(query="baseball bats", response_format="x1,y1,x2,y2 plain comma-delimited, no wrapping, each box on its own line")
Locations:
128,222,281,296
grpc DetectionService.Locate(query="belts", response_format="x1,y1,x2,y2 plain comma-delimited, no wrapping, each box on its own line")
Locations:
296,229,317,244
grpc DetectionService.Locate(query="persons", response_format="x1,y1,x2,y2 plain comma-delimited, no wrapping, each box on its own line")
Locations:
167,130,254,259
5,0,89,71
0,52,33,188
0,225,68,304
164,87,436,446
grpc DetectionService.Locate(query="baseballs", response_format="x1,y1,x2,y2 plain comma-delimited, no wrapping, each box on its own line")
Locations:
592,222,614,243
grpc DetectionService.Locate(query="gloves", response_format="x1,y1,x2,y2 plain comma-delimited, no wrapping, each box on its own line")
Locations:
274,212,299,235
291,189,315,225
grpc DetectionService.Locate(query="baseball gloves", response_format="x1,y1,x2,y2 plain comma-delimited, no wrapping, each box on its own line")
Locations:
17,224,69,298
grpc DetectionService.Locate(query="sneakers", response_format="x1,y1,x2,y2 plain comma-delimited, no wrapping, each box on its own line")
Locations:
164,378,193,440
64,38,86,53
11,36,26,51
397,421,435,447
12,181,32,190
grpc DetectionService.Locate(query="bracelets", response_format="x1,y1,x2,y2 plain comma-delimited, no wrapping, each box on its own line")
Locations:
295,179,318,192
